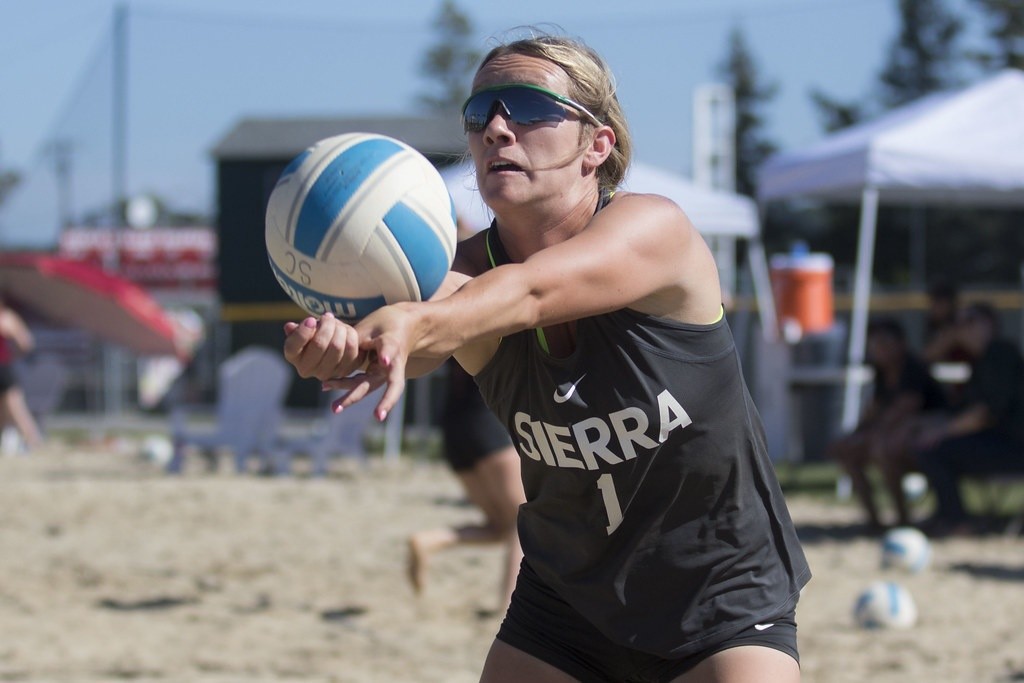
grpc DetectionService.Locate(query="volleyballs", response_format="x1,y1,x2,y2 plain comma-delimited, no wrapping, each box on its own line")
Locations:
855,584,916,631
883,526,930,571
264,131,457,318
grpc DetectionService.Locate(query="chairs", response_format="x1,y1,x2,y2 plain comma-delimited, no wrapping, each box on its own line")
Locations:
279,369,387,479
162,345,297,479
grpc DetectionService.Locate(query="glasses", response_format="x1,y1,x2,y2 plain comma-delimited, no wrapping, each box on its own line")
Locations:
458,81,603,133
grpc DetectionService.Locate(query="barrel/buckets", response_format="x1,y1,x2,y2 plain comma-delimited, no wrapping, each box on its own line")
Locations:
771,253,835,335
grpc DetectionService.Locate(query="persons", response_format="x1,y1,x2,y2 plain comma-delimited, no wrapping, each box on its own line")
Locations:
825,299,1023,539
1,296,51,453
401,217,527,619
279,21,813,682
